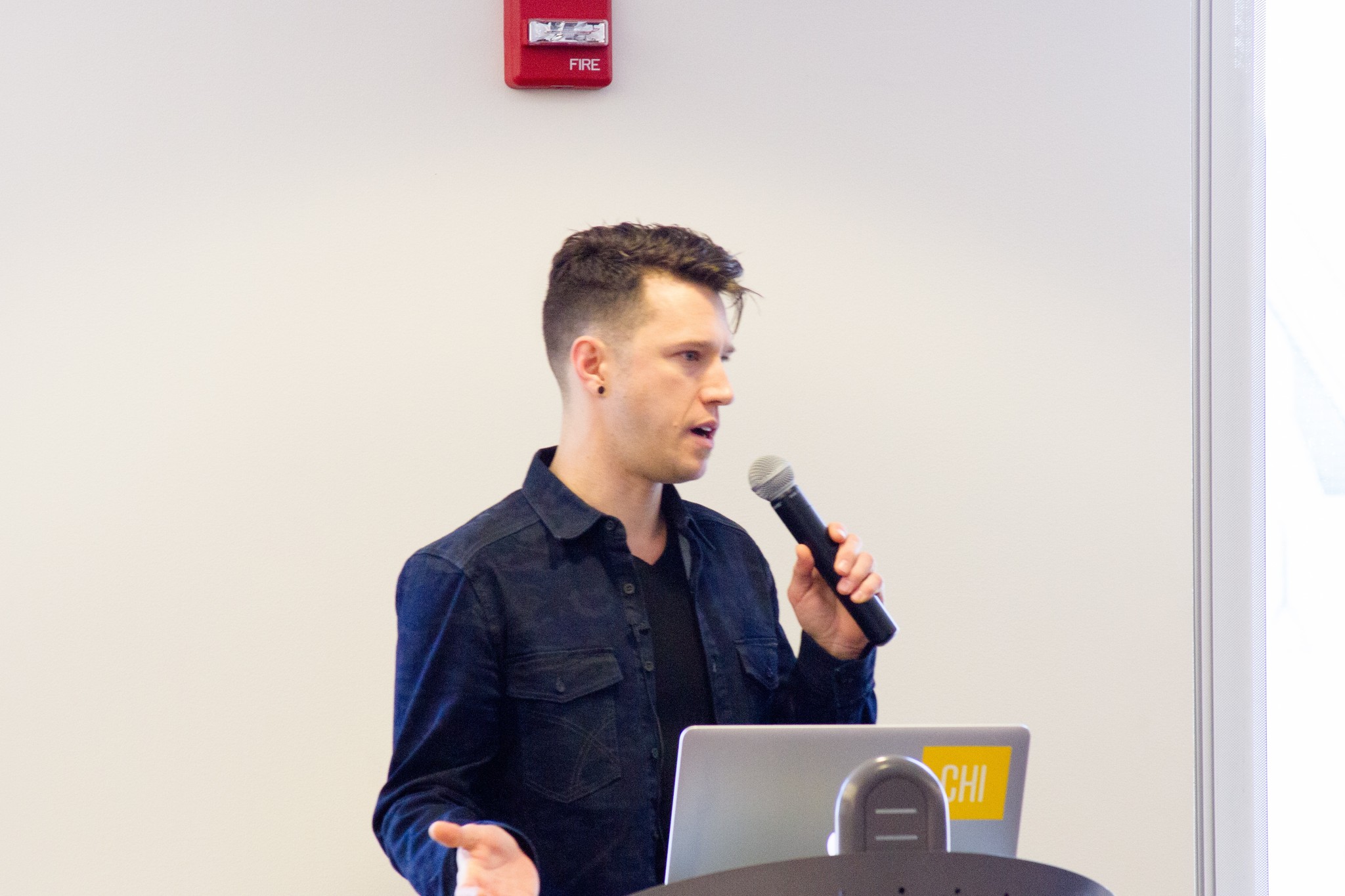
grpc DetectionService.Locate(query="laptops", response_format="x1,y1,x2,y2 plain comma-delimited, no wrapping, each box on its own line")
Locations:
665,726,1032,885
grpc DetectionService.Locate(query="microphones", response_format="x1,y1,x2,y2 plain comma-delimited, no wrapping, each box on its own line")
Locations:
748,453,898,646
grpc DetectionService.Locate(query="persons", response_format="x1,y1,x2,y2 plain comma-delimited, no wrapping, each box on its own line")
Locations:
372,225,885,895
